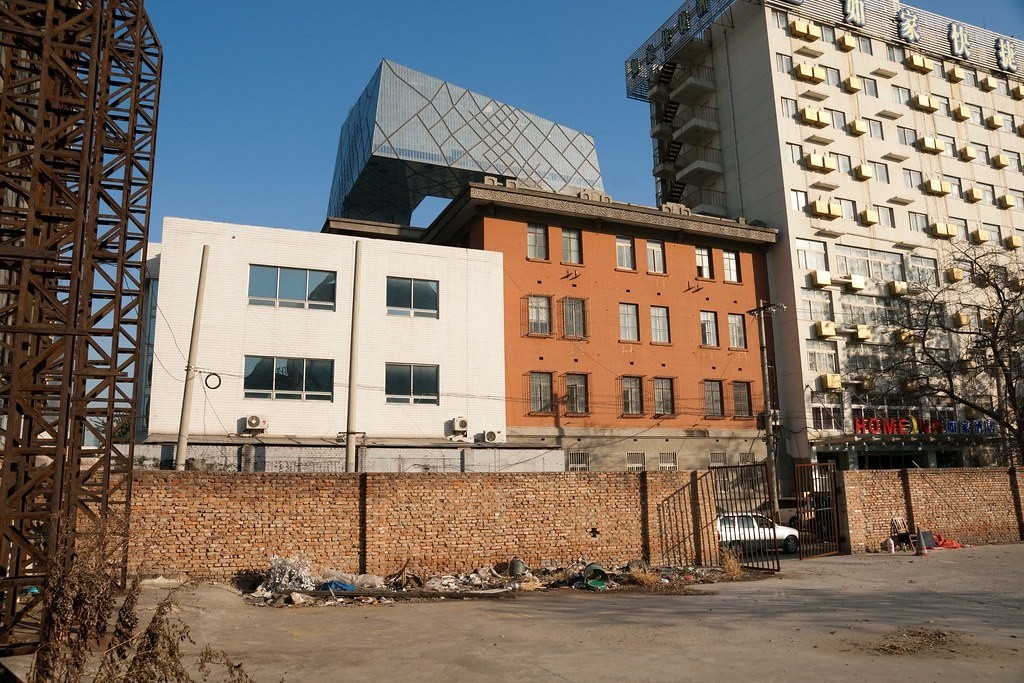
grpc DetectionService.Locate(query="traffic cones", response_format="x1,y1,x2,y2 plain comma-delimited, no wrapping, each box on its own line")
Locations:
912,525,931,556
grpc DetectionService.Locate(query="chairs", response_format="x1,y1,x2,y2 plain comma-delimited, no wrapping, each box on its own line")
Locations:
893,516,916,551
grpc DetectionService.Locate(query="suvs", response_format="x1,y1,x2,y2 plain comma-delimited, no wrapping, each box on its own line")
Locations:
748,497,815,528
716,512,799,558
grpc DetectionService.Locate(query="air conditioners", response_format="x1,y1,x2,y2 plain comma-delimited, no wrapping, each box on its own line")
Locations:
484,429,502,443
246,414,268,430
453,417,468,431
770,410,780,426
